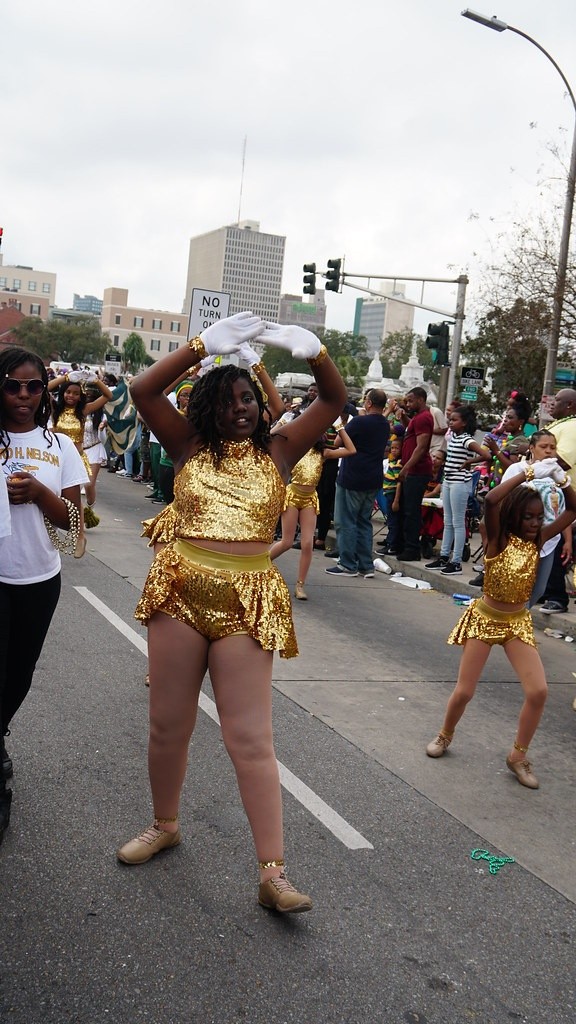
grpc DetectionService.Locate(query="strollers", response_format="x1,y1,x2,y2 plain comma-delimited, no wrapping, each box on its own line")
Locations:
418,461,491,563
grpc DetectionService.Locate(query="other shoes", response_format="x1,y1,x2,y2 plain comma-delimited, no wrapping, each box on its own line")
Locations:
108,466,166,505
74,536,87,558
325,551,339,557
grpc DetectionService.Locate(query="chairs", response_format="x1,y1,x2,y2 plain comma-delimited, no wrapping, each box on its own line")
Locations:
371,469,484,565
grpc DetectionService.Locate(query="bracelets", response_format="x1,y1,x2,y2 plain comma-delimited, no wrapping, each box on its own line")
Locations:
190,335,209,360
188,366,197,376
525,465,534,482
334,424,343,430
252,361,266,375
279,419,287,425
65,372,69,381
307,345,327,366
556,473,568,488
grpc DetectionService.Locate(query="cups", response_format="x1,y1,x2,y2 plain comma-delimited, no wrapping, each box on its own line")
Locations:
373,557,393,574
480,434,495,451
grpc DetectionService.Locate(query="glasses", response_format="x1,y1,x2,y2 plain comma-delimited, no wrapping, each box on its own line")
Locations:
3,378,46,396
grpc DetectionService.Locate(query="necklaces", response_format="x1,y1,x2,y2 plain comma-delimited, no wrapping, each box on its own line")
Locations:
541,415,576,431
43,496,80,556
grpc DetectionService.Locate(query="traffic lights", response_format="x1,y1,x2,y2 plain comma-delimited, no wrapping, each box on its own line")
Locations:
303,262,316,295
325,258,341,292
426,322,450,365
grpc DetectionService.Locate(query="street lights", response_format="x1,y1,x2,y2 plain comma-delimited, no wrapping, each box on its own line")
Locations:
459,7,576,431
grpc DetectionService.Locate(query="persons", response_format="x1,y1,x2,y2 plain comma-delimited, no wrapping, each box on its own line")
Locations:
501,428,572,610
426,457,576,789
119,311,348,913
266,383,532,599
537,388,576,613
0,347,91,773
44,363,194,559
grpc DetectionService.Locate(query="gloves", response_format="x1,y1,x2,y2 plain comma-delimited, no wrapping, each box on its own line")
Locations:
521,457,571,490
278,412,294,426
66,370,98,386
253,320,327,365
189,311,267,358
332,415,345,432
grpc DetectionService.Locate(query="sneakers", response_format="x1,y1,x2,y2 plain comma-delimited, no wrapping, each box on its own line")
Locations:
117,827,181,864
539,602,566,614
506,757,539,789
425,558,450,569
469,573,483,586
472,564,485,571
295,586,307,599
441,563,462,574
0,777,12,843
325,567,357,577
257,872,312,912
427,735,451,757
0,729,13,778
358,569,375,578
374,544,397,556
